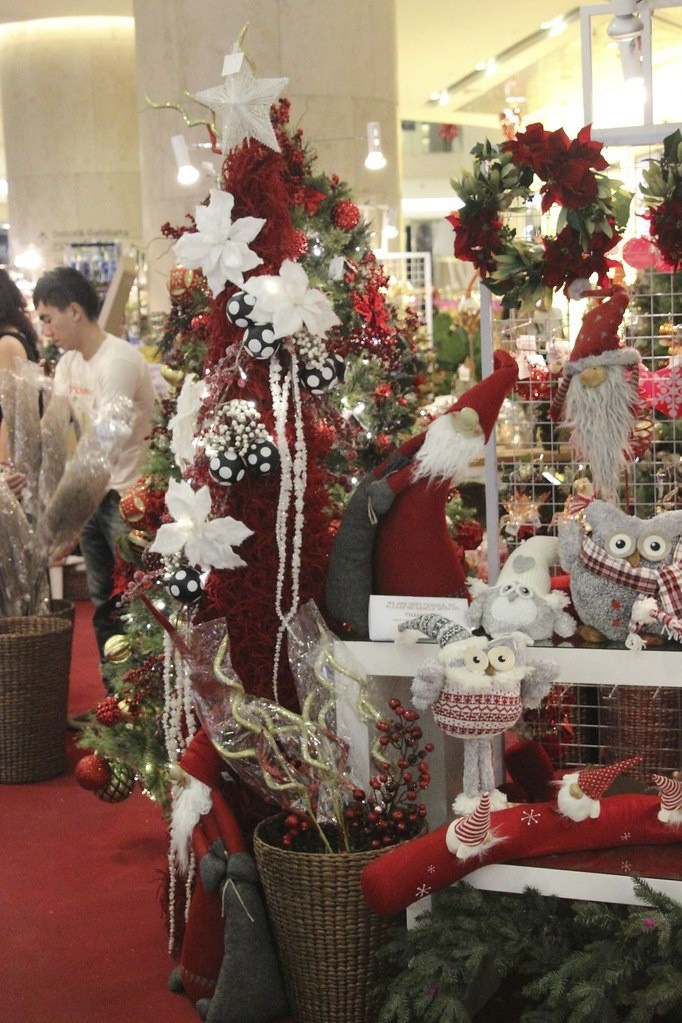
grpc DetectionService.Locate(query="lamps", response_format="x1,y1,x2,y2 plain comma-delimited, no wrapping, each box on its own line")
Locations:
171,134,201,185
364,121,388,170
504,75,527,105
607,0,641,40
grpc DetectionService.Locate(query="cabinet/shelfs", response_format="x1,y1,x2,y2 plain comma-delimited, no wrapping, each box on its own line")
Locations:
333,639,682,936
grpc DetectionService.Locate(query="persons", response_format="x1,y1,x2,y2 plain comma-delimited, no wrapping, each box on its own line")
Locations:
6,266,158,733
0,269,42,479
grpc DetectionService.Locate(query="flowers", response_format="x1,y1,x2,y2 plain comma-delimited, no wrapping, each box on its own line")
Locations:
139,122,682,574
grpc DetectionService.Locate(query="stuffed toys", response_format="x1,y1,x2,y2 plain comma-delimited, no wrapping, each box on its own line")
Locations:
167,722,289,1023
329,291,682,916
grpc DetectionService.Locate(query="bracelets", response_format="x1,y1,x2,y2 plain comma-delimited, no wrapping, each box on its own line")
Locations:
0,462,10,466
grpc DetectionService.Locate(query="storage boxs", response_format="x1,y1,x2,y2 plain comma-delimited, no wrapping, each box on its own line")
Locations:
97,258,136,335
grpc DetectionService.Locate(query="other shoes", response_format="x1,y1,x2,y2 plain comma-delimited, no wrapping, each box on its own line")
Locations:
69,707,115,731
